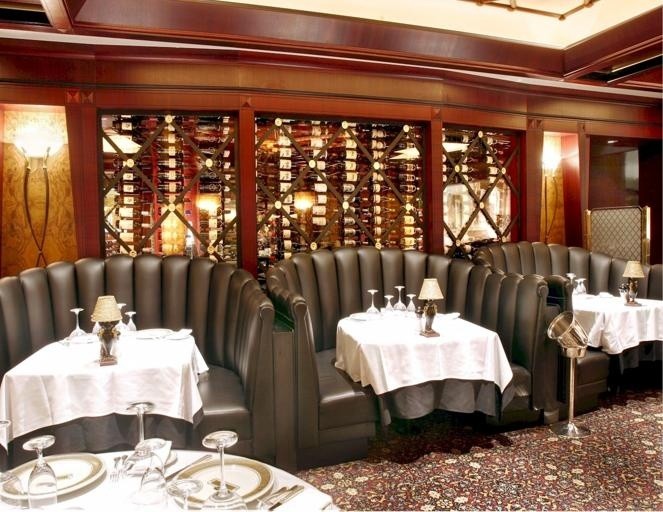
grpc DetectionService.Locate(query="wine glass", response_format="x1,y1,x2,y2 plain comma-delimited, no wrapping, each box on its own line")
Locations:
22,434,62,509
68,308,87,341
120,400,175,509
362,284,416,319
201,430,247,510
124,311,137,336
566,272,589,297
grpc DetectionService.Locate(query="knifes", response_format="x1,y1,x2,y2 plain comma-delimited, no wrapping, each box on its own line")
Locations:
268,486,305,510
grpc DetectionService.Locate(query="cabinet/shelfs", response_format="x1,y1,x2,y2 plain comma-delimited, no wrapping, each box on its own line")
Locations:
97,111,522,292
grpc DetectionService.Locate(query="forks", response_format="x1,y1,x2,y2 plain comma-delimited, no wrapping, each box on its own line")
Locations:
109,447,120,484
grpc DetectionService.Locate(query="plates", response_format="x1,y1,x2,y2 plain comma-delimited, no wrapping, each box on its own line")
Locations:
0,451,106,500
135,328,171,340
351,309,367,321
167,458,272,508
